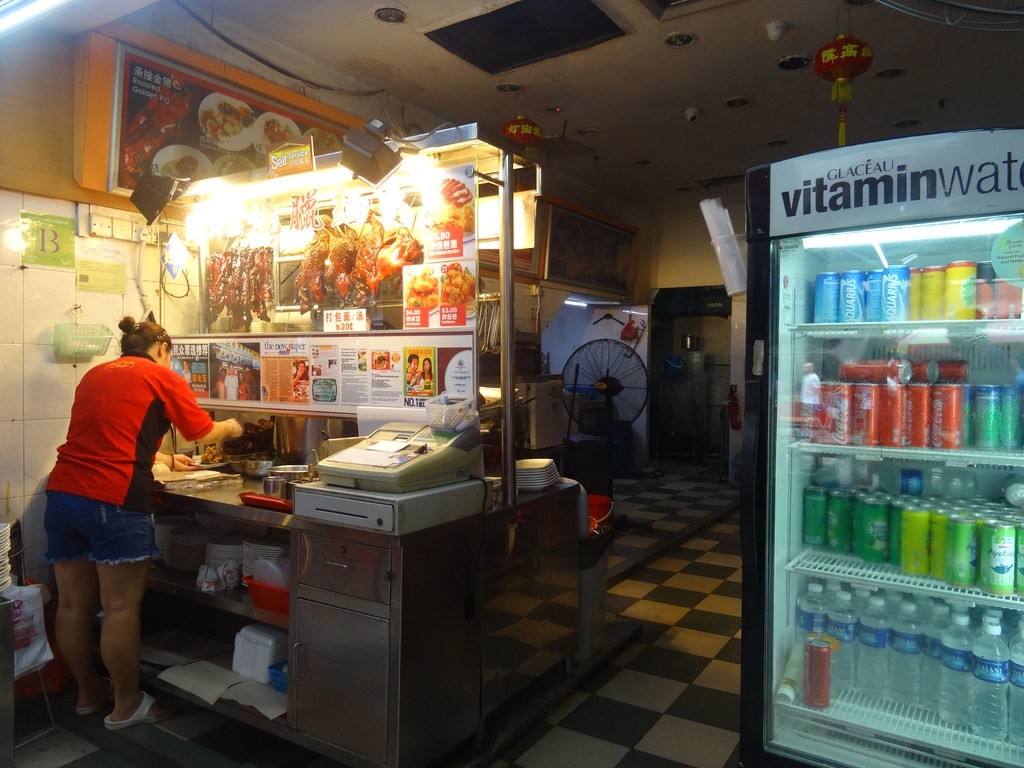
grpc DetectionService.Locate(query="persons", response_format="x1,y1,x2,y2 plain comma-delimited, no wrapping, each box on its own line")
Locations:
406,354,432,391
38,316,246,729
293,360,310,389
800,362,823,437
182,361,257,403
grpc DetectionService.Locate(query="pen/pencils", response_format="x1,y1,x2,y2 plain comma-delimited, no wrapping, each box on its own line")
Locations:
442,394,448,424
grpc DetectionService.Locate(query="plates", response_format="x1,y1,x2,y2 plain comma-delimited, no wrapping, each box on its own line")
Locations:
192,460,231,468
155,516,194,555
0,523,12,593
198,92,341,155
243,536,290,589
168,527,232,574
152,145,258,183
515,459,559,491
205,532,248,590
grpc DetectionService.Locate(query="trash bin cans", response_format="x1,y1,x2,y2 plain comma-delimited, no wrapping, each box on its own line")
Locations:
575,523,617,662
0,597,16,768
563,432,618,494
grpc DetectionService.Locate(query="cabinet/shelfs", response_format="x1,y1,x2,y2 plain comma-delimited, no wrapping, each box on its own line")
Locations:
141,466,580,768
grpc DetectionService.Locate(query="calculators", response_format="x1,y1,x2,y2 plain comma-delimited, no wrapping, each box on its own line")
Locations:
430,398,471,430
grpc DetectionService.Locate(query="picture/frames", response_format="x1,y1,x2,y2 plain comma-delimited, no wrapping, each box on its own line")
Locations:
478,184,545,279
544,204,636,294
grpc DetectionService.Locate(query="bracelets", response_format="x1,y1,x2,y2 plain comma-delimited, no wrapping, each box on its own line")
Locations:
169,453,175,472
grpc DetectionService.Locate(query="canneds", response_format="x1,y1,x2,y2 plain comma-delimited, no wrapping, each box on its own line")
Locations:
809,354,1023,451
813,260,1024,320
803,639,831,708
803,472,1024,600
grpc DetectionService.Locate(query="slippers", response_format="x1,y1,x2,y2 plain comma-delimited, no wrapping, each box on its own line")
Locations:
76,676,115,715
104,690,175,731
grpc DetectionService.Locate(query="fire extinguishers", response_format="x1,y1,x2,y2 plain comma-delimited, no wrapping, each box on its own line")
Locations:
727,384,742,430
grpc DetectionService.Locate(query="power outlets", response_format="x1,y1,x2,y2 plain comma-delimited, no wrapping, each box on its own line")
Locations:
531,284,540,295
132,220,151,244
111,217,132,241
89,212,112,238
151,224,158,245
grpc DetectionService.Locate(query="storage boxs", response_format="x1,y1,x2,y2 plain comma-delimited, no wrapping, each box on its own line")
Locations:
230,620,290,683
268,660,288,695
494,383,580,450
51,322,114,358
242,575,289,617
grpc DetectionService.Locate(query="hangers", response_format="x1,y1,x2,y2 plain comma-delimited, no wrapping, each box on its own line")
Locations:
592,305,625,326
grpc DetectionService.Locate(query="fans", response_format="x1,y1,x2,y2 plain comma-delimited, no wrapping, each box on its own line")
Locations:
561,337,651,533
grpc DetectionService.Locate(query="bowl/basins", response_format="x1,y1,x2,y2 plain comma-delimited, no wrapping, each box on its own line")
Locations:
242,461,272,477
177,449,193,459
264,477,287,500
151,462,170,476
229,460,245,473
289,480,312,501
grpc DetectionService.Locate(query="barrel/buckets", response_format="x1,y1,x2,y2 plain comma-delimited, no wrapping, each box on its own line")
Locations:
665,357,683,374
611,421,633,478
587,495,613,529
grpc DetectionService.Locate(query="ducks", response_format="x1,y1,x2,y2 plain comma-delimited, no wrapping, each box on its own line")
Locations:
294,206,424,315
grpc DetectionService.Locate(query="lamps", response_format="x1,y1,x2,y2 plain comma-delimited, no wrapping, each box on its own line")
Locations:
683,108,701,125
337,116,419,189
132,172,192,226
763,20,788,42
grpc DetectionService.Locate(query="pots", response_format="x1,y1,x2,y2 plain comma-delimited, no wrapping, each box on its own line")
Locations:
273,415,321,465
268,465,309,500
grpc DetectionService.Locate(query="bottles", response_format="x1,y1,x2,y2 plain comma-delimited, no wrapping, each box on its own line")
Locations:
777,642,806,702
796,578,1024,747
807,463,978,497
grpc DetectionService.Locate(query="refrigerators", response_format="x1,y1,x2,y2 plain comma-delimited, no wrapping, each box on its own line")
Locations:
741,128,1024,768
